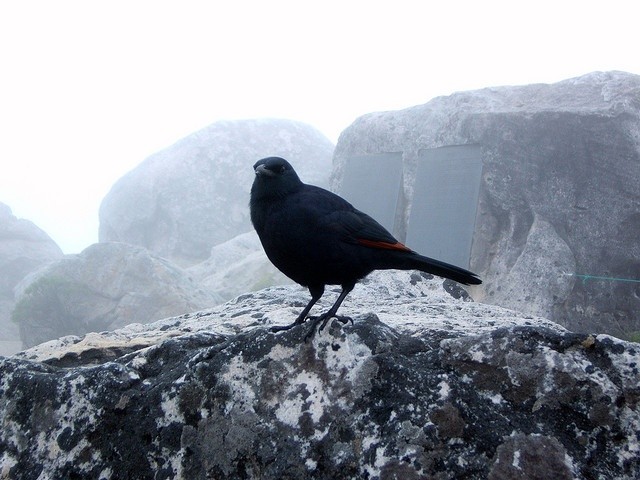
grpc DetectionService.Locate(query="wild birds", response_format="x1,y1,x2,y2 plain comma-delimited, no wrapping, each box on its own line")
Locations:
249,156,483,344
